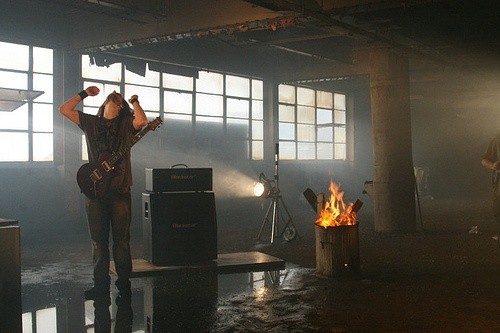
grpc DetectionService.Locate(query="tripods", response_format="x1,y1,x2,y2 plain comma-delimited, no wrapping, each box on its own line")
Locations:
257,143,302,245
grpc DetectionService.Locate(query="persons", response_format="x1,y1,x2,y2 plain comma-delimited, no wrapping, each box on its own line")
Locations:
59,86,147,300
480,132,500,224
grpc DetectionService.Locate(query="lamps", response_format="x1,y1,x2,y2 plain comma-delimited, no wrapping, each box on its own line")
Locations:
253,172,276,199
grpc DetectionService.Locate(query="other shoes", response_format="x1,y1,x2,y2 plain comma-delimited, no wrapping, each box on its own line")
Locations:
117,280,131,290
84,286,109,296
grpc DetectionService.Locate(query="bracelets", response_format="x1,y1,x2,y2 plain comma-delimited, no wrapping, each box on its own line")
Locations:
77,90,88,100
130,98,139,104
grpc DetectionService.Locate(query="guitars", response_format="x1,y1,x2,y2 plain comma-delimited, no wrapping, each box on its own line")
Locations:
76,115,163,201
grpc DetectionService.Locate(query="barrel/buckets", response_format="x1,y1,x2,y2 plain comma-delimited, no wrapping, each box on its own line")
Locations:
314,221,359,277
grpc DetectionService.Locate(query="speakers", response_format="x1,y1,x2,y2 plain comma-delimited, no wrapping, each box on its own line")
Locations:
141,191,218,266
